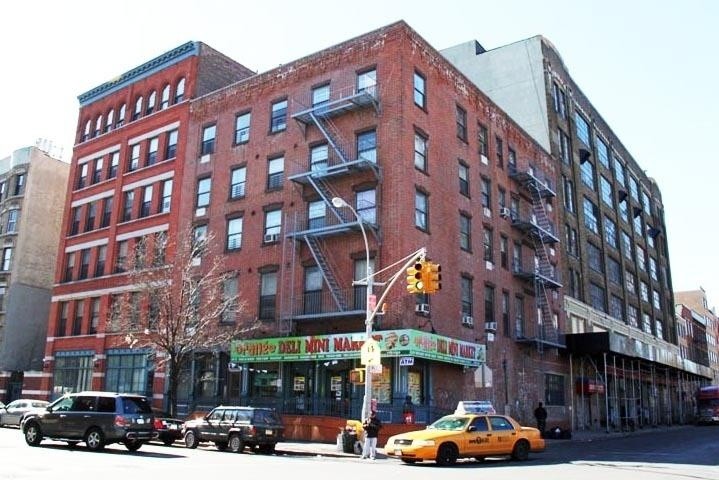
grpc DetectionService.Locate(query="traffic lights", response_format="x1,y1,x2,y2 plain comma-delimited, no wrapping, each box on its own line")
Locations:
406,262,441,295
350,370,363,383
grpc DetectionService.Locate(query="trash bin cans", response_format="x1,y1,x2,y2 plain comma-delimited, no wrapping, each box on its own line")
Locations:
551,428,571,439
343,433,356,453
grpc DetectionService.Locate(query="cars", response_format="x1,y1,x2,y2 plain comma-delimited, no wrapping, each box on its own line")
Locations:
0,399,49,427
383,400,545,465
152,408,184,447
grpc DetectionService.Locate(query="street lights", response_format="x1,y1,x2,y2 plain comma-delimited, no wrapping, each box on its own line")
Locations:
333,197,373,424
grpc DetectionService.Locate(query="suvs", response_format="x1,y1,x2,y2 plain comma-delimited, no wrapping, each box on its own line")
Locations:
182,406,284,453
23,391,159,451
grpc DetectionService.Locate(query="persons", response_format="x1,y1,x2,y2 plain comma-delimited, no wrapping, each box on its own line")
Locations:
359,408,385,460
533,401,547,434
401,394,418,424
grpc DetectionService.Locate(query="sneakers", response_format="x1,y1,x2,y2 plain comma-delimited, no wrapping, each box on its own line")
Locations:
362,455,376,460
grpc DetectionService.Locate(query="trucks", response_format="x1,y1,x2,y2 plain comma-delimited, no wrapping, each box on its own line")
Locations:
691,386,719,425
